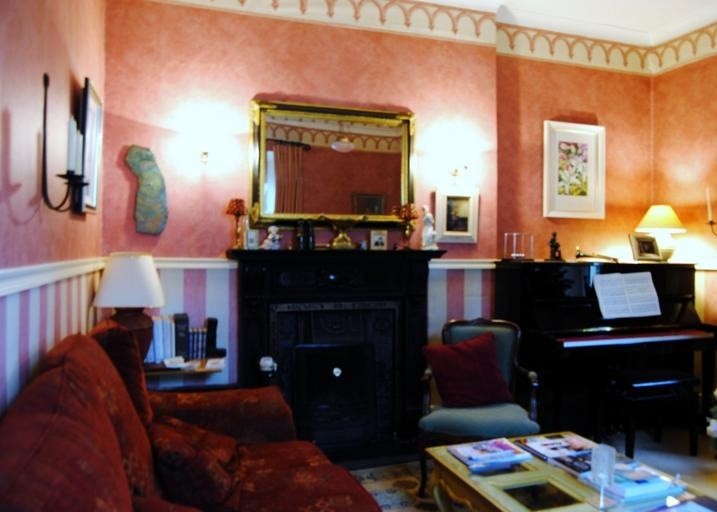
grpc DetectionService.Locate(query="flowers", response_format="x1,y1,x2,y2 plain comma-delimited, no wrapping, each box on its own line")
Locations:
557,140,589,197
391,203,419,221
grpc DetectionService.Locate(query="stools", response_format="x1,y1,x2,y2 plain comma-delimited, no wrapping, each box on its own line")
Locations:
604,371,705,459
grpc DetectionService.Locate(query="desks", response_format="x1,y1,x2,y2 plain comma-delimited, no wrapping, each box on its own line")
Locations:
144,357,283,393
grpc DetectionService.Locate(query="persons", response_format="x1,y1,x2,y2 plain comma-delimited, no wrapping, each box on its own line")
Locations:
375,236,384,246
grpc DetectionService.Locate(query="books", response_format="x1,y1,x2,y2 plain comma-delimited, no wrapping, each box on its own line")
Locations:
594,270,661,319
144,313,217,365
449,431,685,503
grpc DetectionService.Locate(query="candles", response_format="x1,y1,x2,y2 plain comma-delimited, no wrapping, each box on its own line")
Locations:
703,184,712,222
74,130,83,175
65,115,76,172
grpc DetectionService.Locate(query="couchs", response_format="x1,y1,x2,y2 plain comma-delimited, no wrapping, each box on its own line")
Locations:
0,318,383,511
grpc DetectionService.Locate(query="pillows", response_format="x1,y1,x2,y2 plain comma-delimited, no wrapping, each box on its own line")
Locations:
149,414,242,511
422,331,514,408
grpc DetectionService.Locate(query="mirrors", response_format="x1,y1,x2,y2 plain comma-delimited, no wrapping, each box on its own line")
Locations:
245,96,415,230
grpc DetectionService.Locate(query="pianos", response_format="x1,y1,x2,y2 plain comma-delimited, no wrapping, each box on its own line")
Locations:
497,262,716,442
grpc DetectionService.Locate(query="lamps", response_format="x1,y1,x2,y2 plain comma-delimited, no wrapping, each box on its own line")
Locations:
328,120,355,154
224,197,248,250
632,203,687,262
91,250,165,361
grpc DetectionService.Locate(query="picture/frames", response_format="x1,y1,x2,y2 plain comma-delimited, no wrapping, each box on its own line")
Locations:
626,231,662,261
73,77,104,214
433,187,478,245
540,119,605,221
352,192,386,215
370,230,389,251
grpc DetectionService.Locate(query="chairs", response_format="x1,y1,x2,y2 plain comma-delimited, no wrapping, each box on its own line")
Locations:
416,315,539,498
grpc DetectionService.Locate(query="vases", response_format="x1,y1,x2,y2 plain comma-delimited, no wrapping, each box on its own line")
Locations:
401,221,412,250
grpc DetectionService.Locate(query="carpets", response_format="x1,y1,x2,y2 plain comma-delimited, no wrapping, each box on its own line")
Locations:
349,452,447,512
